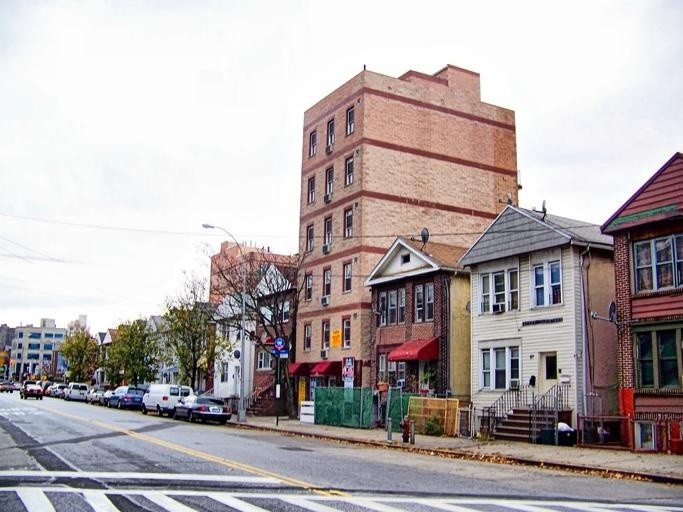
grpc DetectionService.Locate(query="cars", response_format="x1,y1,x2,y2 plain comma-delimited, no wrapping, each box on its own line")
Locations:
0,378,232,423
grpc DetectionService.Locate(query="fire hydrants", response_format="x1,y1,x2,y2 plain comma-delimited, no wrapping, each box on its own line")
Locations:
398,415,411,444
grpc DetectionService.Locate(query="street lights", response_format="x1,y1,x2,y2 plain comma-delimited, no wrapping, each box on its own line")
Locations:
198,223,248,421
